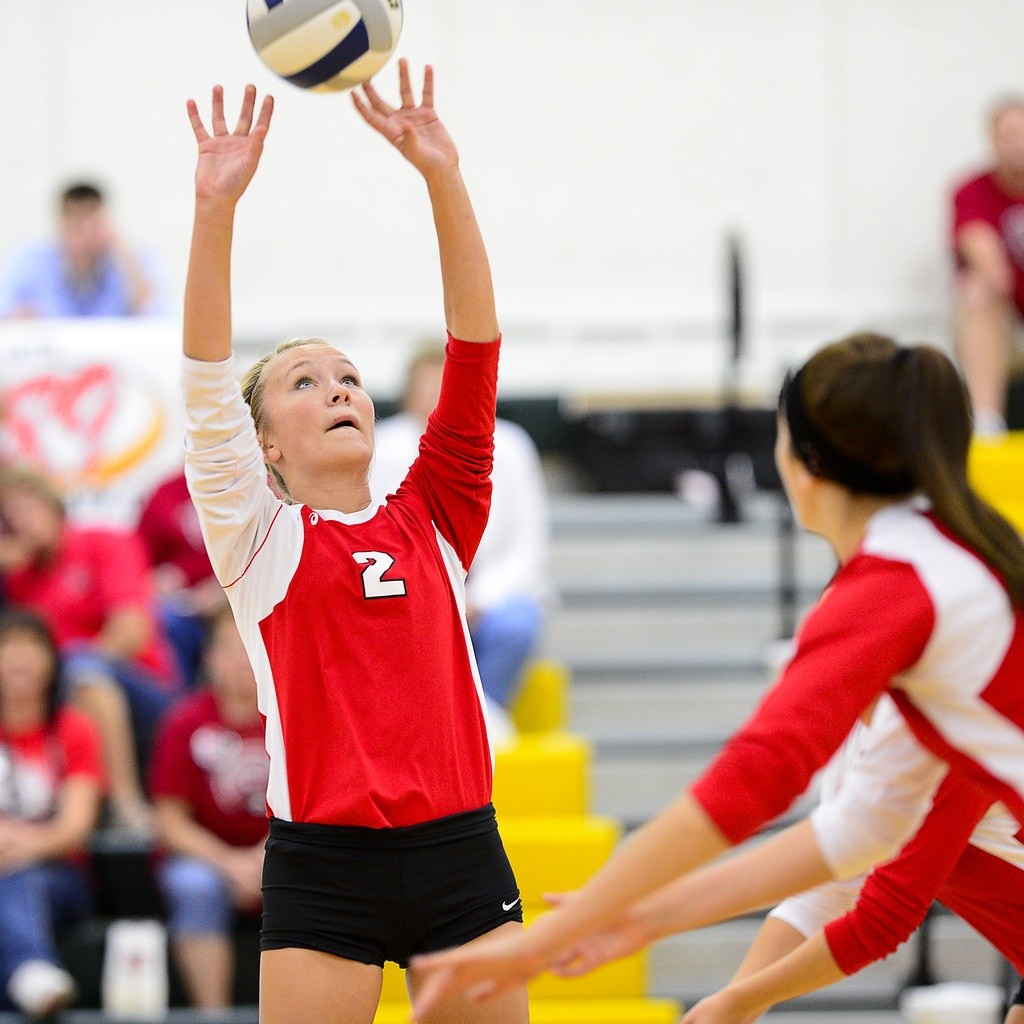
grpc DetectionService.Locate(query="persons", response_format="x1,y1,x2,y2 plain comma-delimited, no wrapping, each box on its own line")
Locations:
177,57,538,1024
1,96,1024,1024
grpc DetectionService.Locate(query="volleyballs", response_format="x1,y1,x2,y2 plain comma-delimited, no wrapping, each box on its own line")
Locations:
245,0,403,95
